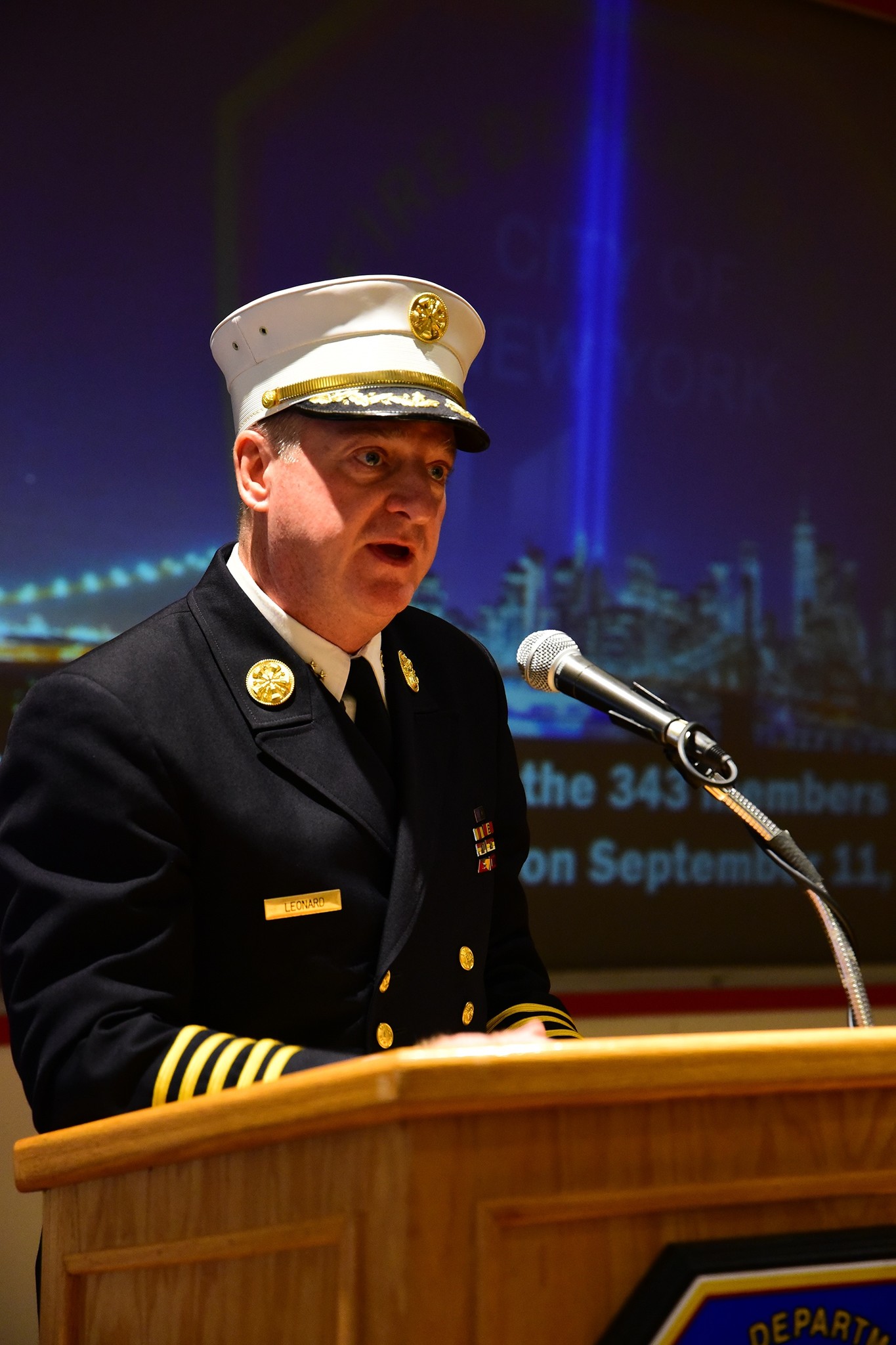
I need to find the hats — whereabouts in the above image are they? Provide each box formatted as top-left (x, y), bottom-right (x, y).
top-left (210, 275), bottom-right (491, 454)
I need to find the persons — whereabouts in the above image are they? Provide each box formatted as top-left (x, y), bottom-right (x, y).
top-left (0, 274), bottom-right (581, 1134)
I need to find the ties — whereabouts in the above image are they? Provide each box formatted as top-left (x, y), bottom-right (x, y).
top-left (345, 656), bottom-right (400, 793)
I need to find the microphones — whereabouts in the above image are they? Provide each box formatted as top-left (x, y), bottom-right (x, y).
top-left (516, 629), bottom-right (731, 769)
